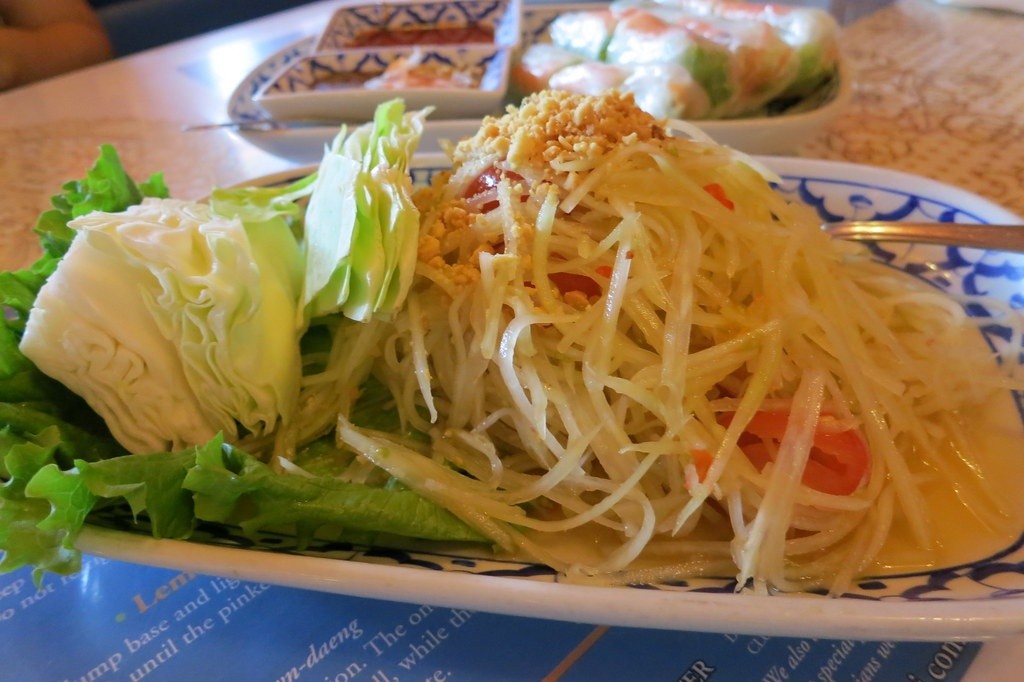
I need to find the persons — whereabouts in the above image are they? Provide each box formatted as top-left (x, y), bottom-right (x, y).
top-left (0, 0), bottom-right (113, 95)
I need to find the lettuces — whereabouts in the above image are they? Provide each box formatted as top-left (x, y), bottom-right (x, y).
top-left (0, 143), bottom-right (508, 588)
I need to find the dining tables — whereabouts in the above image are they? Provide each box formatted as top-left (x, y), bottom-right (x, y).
top-left (0, 0), bottom-right (1024, 681)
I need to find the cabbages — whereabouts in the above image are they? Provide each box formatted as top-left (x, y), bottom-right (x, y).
top-left (18, 97), bottom-right (440, 452)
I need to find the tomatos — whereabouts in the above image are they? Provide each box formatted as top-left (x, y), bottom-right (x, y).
top-left (693, 406), bottom-right (867, 499)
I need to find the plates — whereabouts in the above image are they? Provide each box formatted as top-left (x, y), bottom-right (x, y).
top-left (225, 6), bottom-right (850, 158)
top-left (249, 43), bottom-right (512, 127)
top-left (0, 150), bottom-right (1024, 639)
top-left (312, 1), bottom-right (524, 53)
